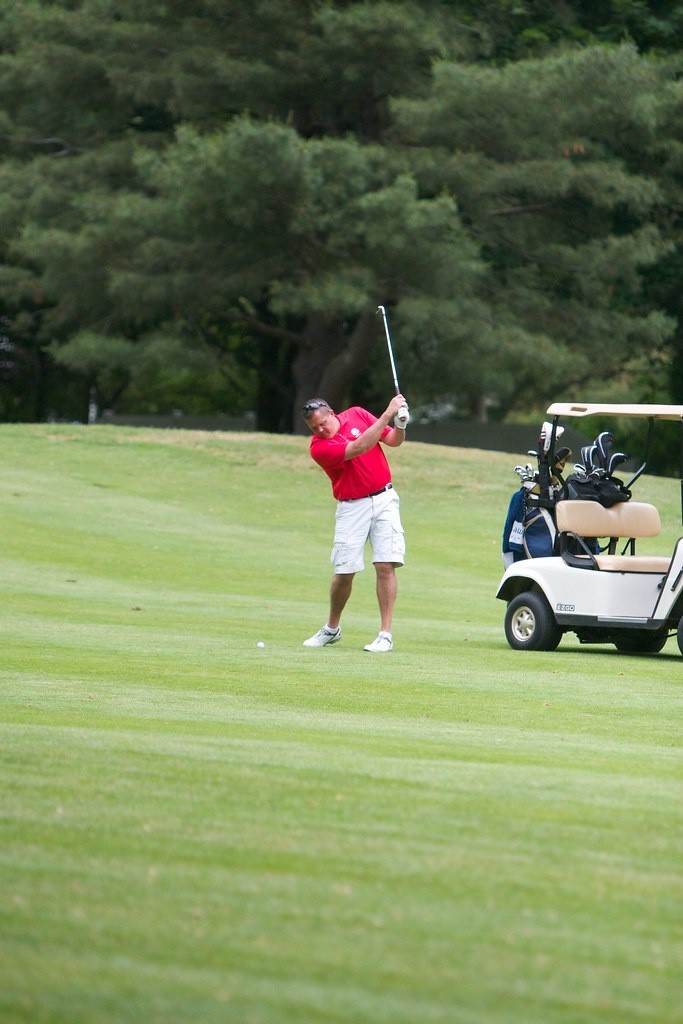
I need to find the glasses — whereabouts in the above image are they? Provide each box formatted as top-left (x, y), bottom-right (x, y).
top-left (301, 402), bottom-right (328, 416)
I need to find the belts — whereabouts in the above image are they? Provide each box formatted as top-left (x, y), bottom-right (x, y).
top-left (338, 484), bottom-right (392, 501)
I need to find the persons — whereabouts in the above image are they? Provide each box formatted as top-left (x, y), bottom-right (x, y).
top-left (301, 394), bottom-right (410, 652)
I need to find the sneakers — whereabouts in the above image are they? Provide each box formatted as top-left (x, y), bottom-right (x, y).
top-left (303, 624), bottom-right (342, 648)
top-left (363, 631), bottom-right (394, 652)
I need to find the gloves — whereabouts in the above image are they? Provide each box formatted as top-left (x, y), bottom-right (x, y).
top-left (394, 402), bottom-right (410, 430)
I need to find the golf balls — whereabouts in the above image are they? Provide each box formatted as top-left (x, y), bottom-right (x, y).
top-left (256, 642), bottom-right (265, 648)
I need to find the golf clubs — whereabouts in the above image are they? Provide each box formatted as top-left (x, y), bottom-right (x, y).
top-left (374, 305), bottom-right (405, 423)
top-left (513, 431), bottom-right (651, 489)
